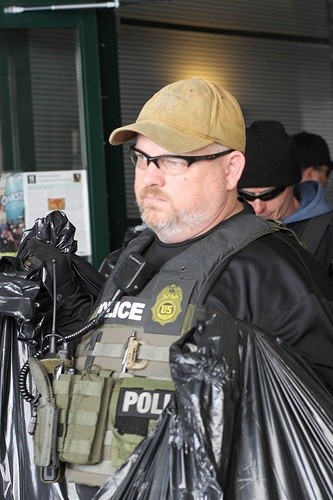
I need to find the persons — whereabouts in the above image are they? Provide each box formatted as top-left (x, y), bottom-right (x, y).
top-left (0, 215), bottom-right (25, 252)
top-left (18, 77), bottom-right (333, 500)
top-left (236, 120), bottom-right (333, 282)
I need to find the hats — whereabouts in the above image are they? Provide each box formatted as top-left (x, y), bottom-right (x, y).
top-left (108, 78), bottom-right (246, 155)
top-left (236, 120), bottom-right (302, 189)
top-left (289, 131), bottom-right (333, 169)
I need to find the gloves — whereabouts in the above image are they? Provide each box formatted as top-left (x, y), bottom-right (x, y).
top-left (26, 238), bottom-right (73, 289)
top-left (194, 304), bottom-right (240, 366)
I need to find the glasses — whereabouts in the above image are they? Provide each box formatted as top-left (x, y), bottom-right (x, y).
top-left (130, 143), bottom-right (236, 175)
top-left (237, 183), bottom-right (288, 201)
top-left (314, 165), bottom-right (331, 178)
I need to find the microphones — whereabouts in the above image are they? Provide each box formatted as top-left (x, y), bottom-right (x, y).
top-left (112, 251), bottom-right (152, 296)
